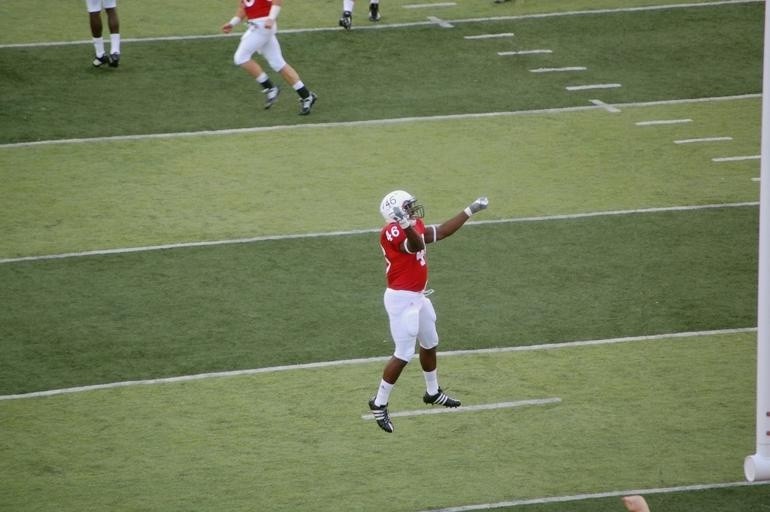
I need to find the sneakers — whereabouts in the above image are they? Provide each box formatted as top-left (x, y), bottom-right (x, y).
top-left (91, 53), bottom-right (119, 69)
top-left (370, 1), bottom-right (380, 21)
top-left (262, 86), bottom-right (279, 109)
top-left (423, 386), bottom-right (460, 408)
top-left (368, 396), bottom-right (394, 433)
top-left (339, 15), bottom-right (351, 30)
top-left (299, 92), bottom-right (317, 115)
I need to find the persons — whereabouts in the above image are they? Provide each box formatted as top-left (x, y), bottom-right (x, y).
top-left (621, 494), bottom-right (650, 512)
top-left (85, 0), bottom-right (121, 69)
top-left (338, 0), bottom-right (382, 31)
top-left (222, 1), bottom-right (319, 117)
top-left (368, 190), bottom-right (490, 432)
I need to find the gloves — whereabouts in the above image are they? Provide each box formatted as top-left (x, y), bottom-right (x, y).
top-left (393, 207), bottom-right (411, 229)
top-left (465, 198), bottom-right (488, 216)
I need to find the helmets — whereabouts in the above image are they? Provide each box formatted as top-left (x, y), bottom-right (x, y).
top-left (380, 190), bottom-right (424, 225)
